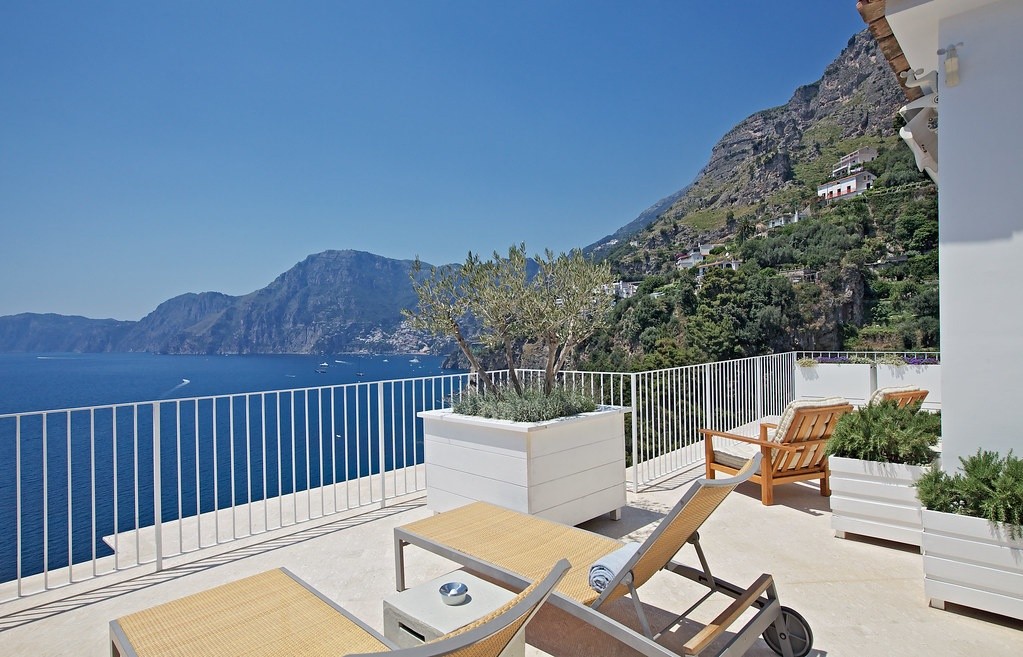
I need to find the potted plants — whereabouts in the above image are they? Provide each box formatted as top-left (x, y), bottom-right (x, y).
top-left (823, 397), bottom-right (941, 547)
top-left (911, 441), bottom-right (1023, 620)
top-left (399, 241), bottom-right (643, 529)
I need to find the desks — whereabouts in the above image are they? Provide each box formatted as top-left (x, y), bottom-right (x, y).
top-left (383, 569), bottom-right (525, 657)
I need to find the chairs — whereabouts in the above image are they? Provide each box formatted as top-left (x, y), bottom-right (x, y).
top-left (699, 396), bottom-right (854, 505)
top-left (868, 383), bottom-right (930, 414)
top-left (108, 452), bottom-right (814, 657)
